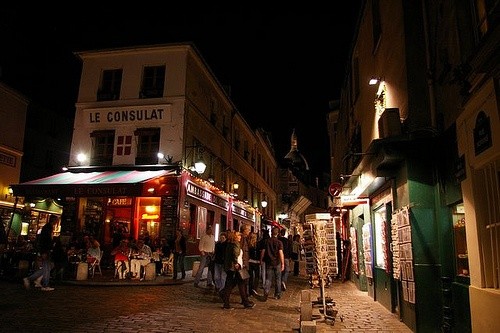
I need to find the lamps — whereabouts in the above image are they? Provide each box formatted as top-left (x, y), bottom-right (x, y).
top-left (368, 77), bottom-right (385, 85)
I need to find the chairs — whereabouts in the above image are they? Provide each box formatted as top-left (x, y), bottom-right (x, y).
top-left (89, 251), bottom-right (103, 279)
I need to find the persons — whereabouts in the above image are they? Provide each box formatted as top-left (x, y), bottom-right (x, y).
top-left (194, 222), bottom-right (302, 309)
top-left (0, 217), bottom-right (54, 288)
top-left (173, 229), bottom-right (187, 280)
top-left (49, 231), bottom-right (101, 278)
top-left (24, 215), bottom-right (59, 292)
top-left (110, 226), bottom-right (170, 280)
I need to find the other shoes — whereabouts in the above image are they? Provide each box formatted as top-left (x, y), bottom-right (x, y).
top-left (41, 287), bottom-right (54, 291)
top-left (251, 286), bottom-right (259, 295)
top-left (263, 291), bottom-right (269, 301)
top-left (245, 303), bottom-right (256, 309)
top-left (223, 305), bottom-right (234, 311)
top-left (194, 282), bottom-right (198, 286)
top-left (34, 281), bottom-right (42, 288)
top-left (207, 283), bottom-right (213, 287)
top-left (24, 278), bottom-right (29, 288)
top-left (281, 281), bottom-right (286, 291)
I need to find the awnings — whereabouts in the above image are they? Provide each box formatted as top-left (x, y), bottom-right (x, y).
top-left (8, 168), bottom-right (176, 197)
